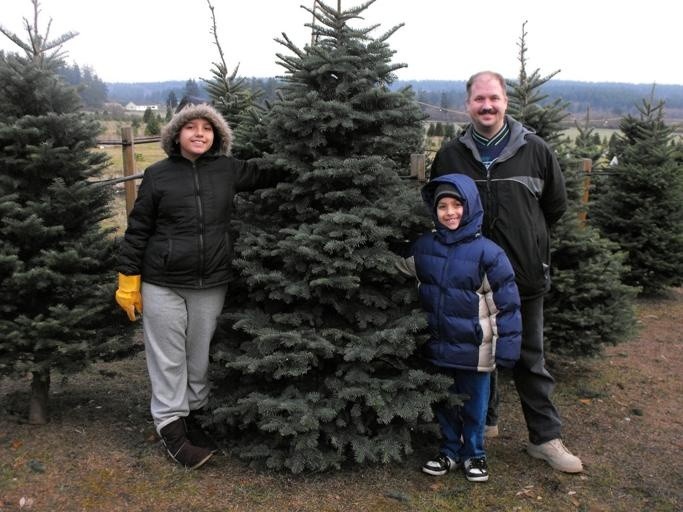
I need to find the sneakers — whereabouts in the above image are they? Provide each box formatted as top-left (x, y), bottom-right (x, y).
top-left (463, 455), bottom-right (490, 482)
top-left (526, 439), bottom-right (583, 474)
top-left (421, 455), bottom-right (461, 476)
top-left (460, 424), bottom-right (500, 445)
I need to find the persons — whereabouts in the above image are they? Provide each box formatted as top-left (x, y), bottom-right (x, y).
top-left (112, 97), bottom-right (290, 470)
top-left (428, 70), bottom-right (585, 476)
top-left (382, 173), bottom-right (522, 484)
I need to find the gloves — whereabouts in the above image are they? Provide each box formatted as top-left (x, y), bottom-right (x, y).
top-left (115, 270), bottom-right (143, 322)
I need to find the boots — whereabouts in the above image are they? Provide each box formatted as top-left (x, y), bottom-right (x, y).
top-left (155, 407), bottom-right (222, 471)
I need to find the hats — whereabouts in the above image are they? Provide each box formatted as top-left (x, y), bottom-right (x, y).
top-left (433, 182), bottom-right (464, 209)
